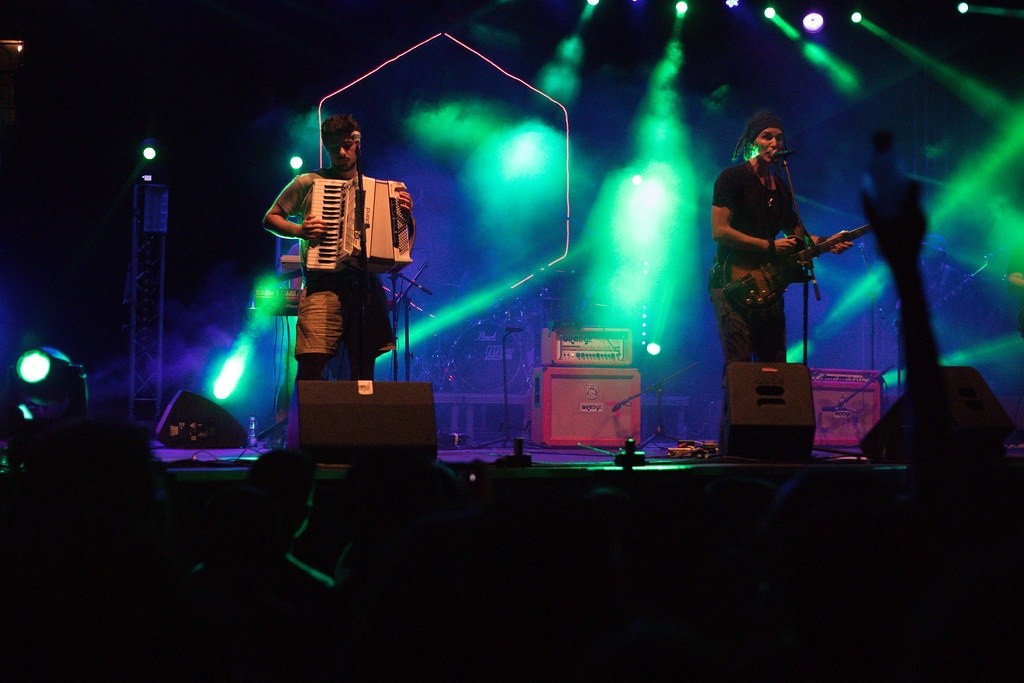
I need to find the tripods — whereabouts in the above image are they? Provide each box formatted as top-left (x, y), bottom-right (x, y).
top-left (620, 360), bottom-right (700, 452)
top-left (472, 326), bottom-right (555, 449)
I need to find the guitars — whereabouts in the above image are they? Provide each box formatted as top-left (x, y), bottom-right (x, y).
top-left (720, 223), bottom-right (873, 308)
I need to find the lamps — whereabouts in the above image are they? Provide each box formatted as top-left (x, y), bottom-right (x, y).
top-left (139, 138), bottom-right (164, 162)
top-left (7, 346), bottom-right (89, 417)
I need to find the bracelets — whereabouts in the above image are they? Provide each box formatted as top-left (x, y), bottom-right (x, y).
top-left (767, 238), bottom-right (775, 252)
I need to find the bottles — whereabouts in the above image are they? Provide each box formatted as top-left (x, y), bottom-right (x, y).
top-left (247, 416), bottom-right (257, 447)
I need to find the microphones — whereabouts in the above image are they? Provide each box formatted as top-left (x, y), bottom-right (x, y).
top-left (821, 405), bottom-right (848, 411)
top-left (612, 404), bottom-right (621, 412)
top-left (351, 130), bottom-right (361, 144)
top-left (396, 263), bottom-right (429, 305)
top-left (769, 149), bottom-right (796, 160)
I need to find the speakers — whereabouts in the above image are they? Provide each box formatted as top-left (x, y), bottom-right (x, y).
top-left (859, 366), bottom-right (1014, 465)
top-left (529, 365), bottom-right (642, 450)
top-left (809, 367), bottom-right (883, 446)
top-left (286, 380), bottom-right (438, 463)
top-left (154, 390), bottom-right (247, 449)
top-left (719, 362), bottom-right (817, 462)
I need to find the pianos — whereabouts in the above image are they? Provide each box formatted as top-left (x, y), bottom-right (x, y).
top-left (252, 286), bottom-right (405, 443)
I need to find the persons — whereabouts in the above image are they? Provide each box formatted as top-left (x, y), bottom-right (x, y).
top-left (707, 113), bottom-right (855, 459)
top-left (262, 114), bottom-right (417, 381)
